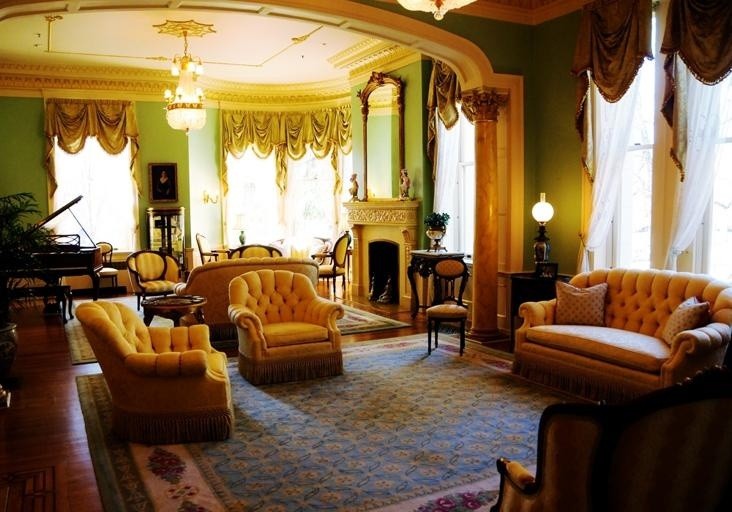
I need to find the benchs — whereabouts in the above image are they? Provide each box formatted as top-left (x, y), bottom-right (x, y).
top-left (7, 285), bottom-right (74, 324)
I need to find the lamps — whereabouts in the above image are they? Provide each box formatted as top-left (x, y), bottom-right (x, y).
top-left (532, 192), bottom-right (554, 279)
top-left (163, 27), bottom-right (207, 135)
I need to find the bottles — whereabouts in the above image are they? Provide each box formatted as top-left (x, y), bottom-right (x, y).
top-left (239, 231), bottom-right (245, 246)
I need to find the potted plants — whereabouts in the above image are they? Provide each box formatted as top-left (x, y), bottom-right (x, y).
top-left (424, 212), bottom-right (450, 239)
top-left (0, 191), bottom-right (62, 375)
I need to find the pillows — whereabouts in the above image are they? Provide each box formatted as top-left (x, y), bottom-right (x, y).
top-left (662, 296), bottom-right (710, 349)
top-left (555, 280), bottom-right (608, 326)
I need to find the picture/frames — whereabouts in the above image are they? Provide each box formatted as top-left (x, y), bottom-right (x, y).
top-left (149, 163), bottom-right (178, 203)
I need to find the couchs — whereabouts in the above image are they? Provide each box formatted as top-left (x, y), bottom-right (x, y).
top-left (511, 267), bottom-right (732, 400)
top-left (174, 257), bottom-right (319, 338)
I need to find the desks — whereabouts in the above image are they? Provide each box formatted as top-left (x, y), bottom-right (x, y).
top-left (407, 250), bottom-right (468, 319)
top-left (141, 295), bottom-right (208, 326)
top-left (499, 272), bottom-right (573, 346)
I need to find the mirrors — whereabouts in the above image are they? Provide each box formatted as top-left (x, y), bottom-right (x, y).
top-left (357, 72), bottom-right (407, 200)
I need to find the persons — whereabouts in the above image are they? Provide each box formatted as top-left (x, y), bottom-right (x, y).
top-left (349, 173), bottom-right (358, 201)
top-left (398, 168), bottom-right (411, 198)
top-left (154, 168), bottom-right (174, 199)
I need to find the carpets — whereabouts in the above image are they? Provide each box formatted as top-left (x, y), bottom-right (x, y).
top-left (76, 331), bottom-right (590, 512)
top-left (59, 295), bottom-right (411, 365)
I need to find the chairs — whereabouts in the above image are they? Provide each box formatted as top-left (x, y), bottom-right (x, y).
top-left (74, 300), bottom-right (234, 445)
top-left (196, 233), bottom-right (351, 292)
top-left (426, 256), bottom-right (470, 356)
top-left (490, 365), bottom-right (731, 512)
top-left (95, 242), bottom-right (118, 300)
top-left (125, 250), bottom-right (181, 311)
top-left (228, 269), bottom-right (344, 385)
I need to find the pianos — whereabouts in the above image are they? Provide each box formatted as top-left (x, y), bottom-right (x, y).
top-left (1, 194), bottom-right (104, 301)
